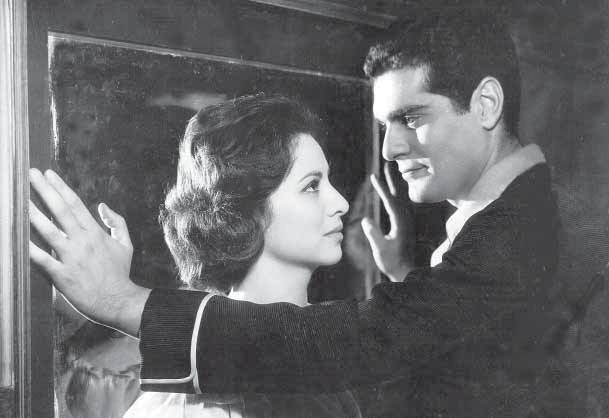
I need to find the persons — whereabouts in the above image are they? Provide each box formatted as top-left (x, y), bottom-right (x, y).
top-left (111, 91), bottom-right (350, 418)
top-left (27, 1), bottom-right (609, 417)
top-left (48, 99), bottom-right (359, 415)
